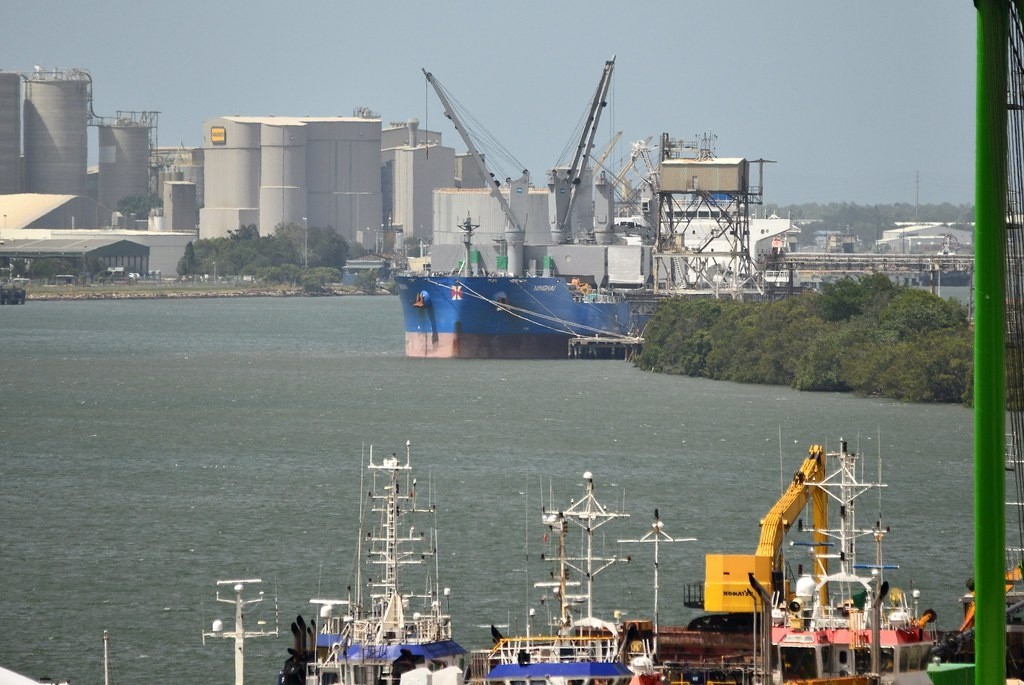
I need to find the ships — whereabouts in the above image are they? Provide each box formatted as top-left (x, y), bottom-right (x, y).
top-left (393, 49), bottom-right (788, 360)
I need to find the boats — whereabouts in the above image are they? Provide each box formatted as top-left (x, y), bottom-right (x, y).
top-left (214, 430), bottom-right (1024, 685)
